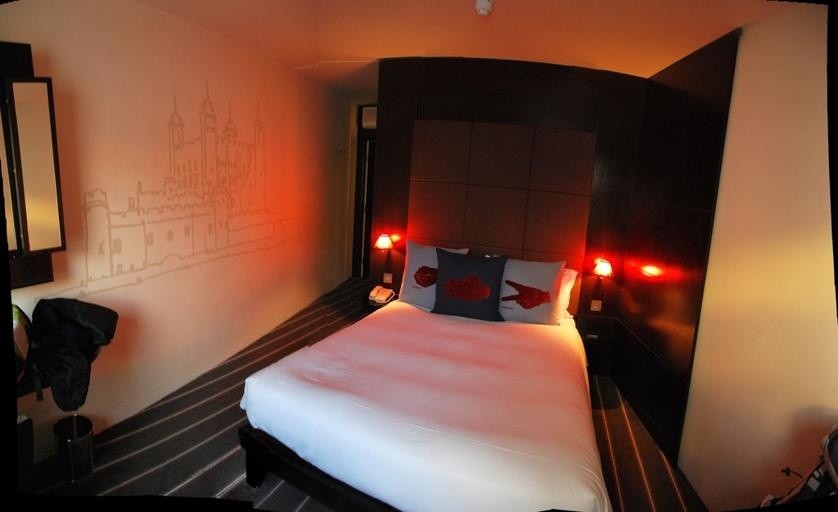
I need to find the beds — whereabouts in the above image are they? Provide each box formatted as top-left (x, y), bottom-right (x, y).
top-left (238, 298), bottom-right (613, 512)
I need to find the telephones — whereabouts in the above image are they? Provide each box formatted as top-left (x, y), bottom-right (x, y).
top-left (369, 285), bottom-right (395, 303)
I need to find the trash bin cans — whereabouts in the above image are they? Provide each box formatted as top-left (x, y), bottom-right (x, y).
top-left (53, 414), bottom-right (95, 479)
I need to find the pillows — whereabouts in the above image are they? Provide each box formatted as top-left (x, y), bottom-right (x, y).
top-left (398, 240), bottom-right (578, 326)
